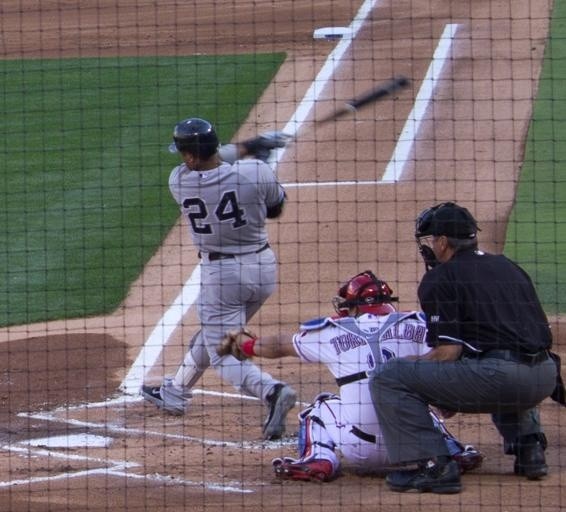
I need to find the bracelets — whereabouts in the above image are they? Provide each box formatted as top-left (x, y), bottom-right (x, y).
top-left (242, 339), bottom-right (256, 357)
top-left (215, 143), bottom-right (238, 164)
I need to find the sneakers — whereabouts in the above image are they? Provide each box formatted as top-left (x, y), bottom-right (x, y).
top-left (385, 460), bottom-right (461, 492)
top-left (263, 384), bottom-right (296, 439)
top-left (275, 459), bottom-right (332, 483)
top-left (139, 385), bottom-right (184, 416)
top-left (514, 444), bottom-right (547, 477)
top-left (454, 449), bottom-right (483, 473)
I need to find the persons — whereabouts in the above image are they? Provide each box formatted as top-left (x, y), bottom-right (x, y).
top-left (368, 202), bottom-right (557, 493)
top-left (218, 271), bottom-right (484, 481)
top-left (138, 118), bottom-right (297, 441)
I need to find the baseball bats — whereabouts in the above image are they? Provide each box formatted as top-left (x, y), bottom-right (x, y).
top-left (292, 76), bottom-right (409, 140)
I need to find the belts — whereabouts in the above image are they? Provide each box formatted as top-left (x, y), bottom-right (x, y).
top-left (351, 426), bottom-right (375, 443)
top-left (485, 352), bottom-right (548, 362)
top-left (199, 243), bottom-right (269, 259)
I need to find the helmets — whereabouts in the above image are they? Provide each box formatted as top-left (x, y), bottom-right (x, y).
top-left (331, 271), bottom-right (398, 316)
top-left (168, 118), bottom-right (218, 153)
top-left (414, 201), bottom-right (481, 271)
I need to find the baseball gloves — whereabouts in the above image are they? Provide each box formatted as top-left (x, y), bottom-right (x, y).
top-left (216, 329), bottom-right (257, 361)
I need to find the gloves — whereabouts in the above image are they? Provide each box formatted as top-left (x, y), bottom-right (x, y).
top-left (245, 132), bottom-right (291, 160)
